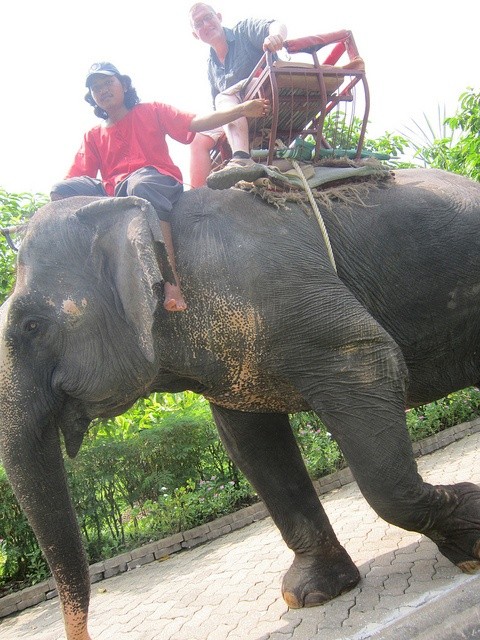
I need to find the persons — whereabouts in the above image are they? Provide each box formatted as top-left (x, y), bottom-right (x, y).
top-left (188, 2), bottom-right (287, 190)
top-left (50, 62), bottom-right (272, 311)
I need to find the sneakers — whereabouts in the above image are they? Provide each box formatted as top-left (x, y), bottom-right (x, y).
top-left (205, 157), bottom-right (264, 190)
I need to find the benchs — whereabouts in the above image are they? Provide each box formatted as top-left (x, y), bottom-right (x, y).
top-left (208, 30), bottom-right (372, 172)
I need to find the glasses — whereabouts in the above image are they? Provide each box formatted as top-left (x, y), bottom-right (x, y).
top-left (192, 13), bottom-right (213, 29)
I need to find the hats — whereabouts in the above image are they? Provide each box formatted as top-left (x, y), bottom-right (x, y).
top-left (86, 62), bottom-right (121, 88)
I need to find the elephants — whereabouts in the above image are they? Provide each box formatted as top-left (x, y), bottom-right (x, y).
top-left (0, 167), bottom-right (480, 640)
